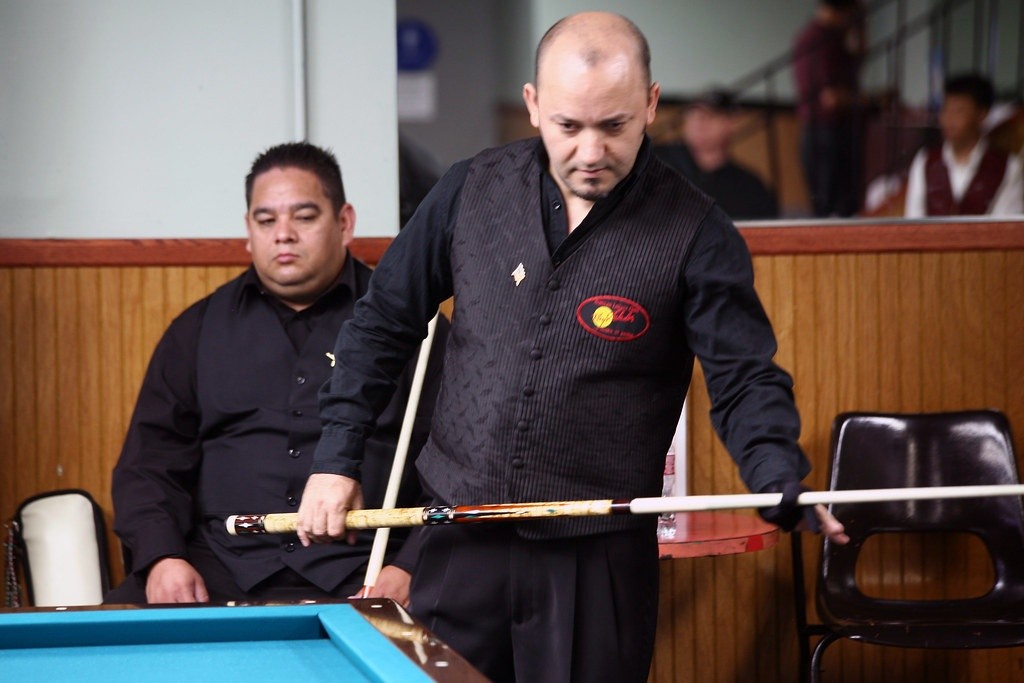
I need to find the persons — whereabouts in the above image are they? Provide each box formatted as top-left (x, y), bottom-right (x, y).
top-left (98, 143), bottom-right (452, 613)
top-left (863, 70), bottom-right (1024, 220)
top-left (659, 91), bottom-right (780, 220)
top-left (793, 0), bottom-right (885, 218)
top-left (294, 12), bottom-right (850, 683)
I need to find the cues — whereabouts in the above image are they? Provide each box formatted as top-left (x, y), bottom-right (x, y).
top-left (359, 306), bottom-right (442, 598)
top-left (223, 483), bottom-right (1024, 537)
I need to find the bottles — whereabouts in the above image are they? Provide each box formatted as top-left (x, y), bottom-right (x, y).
top-left (658, 439), bottom-right (675, 522)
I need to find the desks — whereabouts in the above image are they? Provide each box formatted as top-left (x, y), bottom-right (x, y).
top-left (658, 511), bottom-right (782, 560)
top-left (0, 597), bottom-right (491, 683)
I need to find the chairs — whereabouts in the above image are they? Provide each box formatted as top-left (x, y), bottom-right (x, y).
top-left (807, 407), bottom-right (1024, 683)
